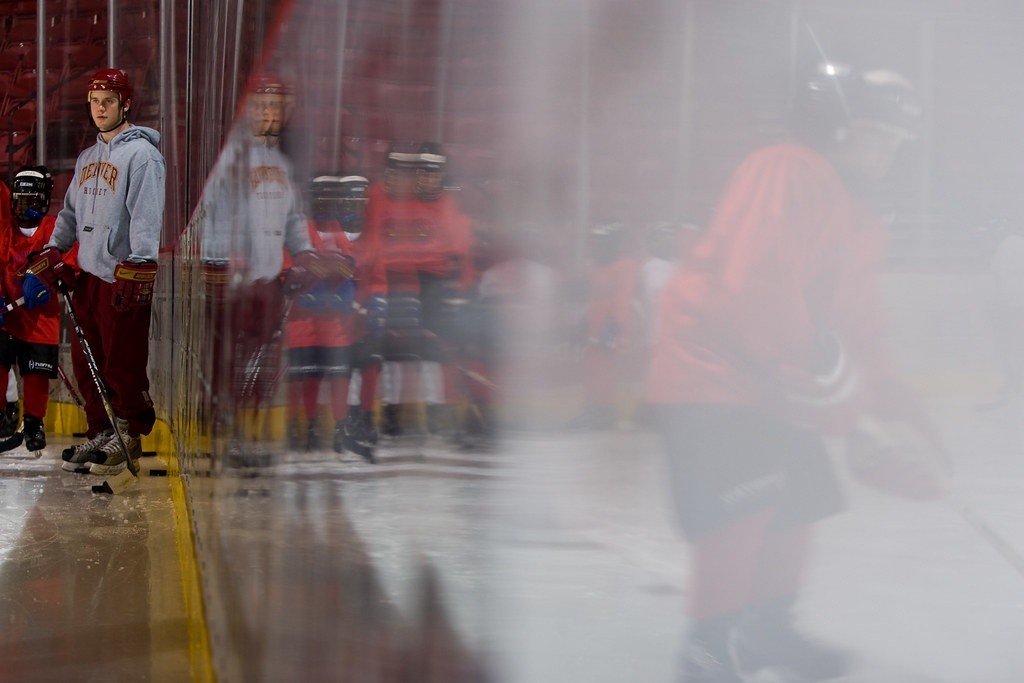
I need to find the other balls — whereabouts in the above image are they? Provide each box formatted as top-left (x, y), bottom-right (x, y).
top-left (72, 433), bottom-right (86, 437)
top-left (141, 451), bottom-right (157, 457)
top-left (74, 466), bottom-right (90, 474)
top-left (92, 485), bottom-right (105, 493)
top-left (150, 470), bottom-right (167, 476)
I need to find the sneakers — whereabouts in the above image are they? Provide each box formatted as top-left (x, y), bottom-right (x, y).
top-left (23, 414), bottom-right (47, 459)
top-left (61, 426), bottom-right (117, 472)
top-left (0, 403), bottom-right (24, 453)
top-left (88, 416), bottom-right (143, 476)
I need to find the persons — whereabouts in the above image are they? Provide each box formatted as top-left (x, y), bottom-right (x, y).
top-left (367, 140), bottom-right (497, 465)
top-left (27, 66), bottom-right (169, 476)
top-left (287, 166), bottom-right (385, 463)
top-left (0, 165), bottom-right (82, 452)
top-left (641, 61), bottom-right (961, 680)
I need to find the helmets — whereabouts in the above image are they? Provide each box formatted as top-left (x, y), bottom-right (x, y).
top-left (243, 66), bottom-right (287, 96)
top-left (86, 67), bottom-right (134, 107)
top-left (10, 165), bottom-right (55, 227)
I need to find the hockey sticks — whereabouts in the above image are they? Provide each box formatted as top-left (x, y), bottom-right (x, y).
top-left (55, 276), bottom-right (139, 495)
top-left (0, 289), bottom-right (83, 408)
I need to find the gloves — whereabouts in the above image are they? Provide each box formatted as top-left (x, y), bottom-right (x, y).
top-left (22, 269), bottom-right (50, 309)
top-left (0, 295), bottom-right (8, 326)
top-left (110, 260), bottom-right (159, 317)
top-left (27, 246), bottom-right (78, 293)
